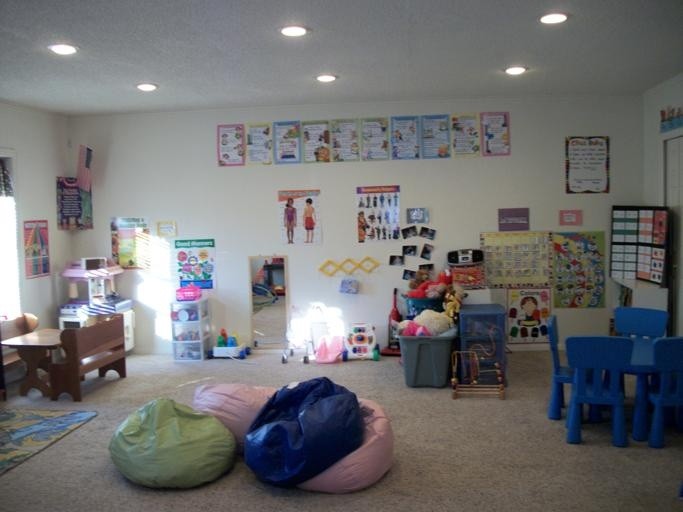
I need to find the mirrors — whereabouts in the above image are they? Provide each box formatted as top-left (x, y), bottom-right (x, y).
top-left (248, 255), bottom-right (289, 350)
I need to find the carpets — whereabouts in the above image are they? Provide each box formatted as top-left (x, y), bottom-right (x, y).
top-left (1, 410), bottom-right (99, 478)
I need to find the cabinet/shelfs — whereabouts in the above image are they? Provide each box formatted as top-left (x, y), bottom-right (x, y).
top-left (170, 295), bottom-right (210, 362)
top-left (459, 304), bottom-right (507, 384)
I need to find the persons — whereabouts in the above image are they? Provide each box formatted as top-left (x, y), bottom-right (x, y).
top-left (357, 210), bottom-right (369, 243)
top-left (301, 197), bottom-right (318, 244)
top-left (281, 196), bottom-right (298, 244)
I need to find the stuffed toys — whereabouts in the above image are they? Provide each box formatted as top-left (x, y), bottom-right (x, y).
top-left (397, 310), bottom-right (456, 339)
top-left (403, 267), bottom-right (453, 300)
top-left (408, 268), bottom-right (430, 290)
top-left (442, 287), bottom-right (468, 319)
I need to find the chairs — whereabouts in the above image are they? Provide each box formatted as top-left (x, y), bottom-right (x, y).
top-left (546, 307), bottom-right (682, 449)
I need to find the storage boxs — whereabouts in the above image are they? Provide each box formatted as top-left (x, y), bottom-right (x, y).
top-left (397, 336), bottom-right (454, 388)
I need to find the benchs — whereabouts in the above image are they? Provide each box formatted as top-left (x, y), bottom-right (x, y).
top-left (0, 313), bottom-right (126, 402)
